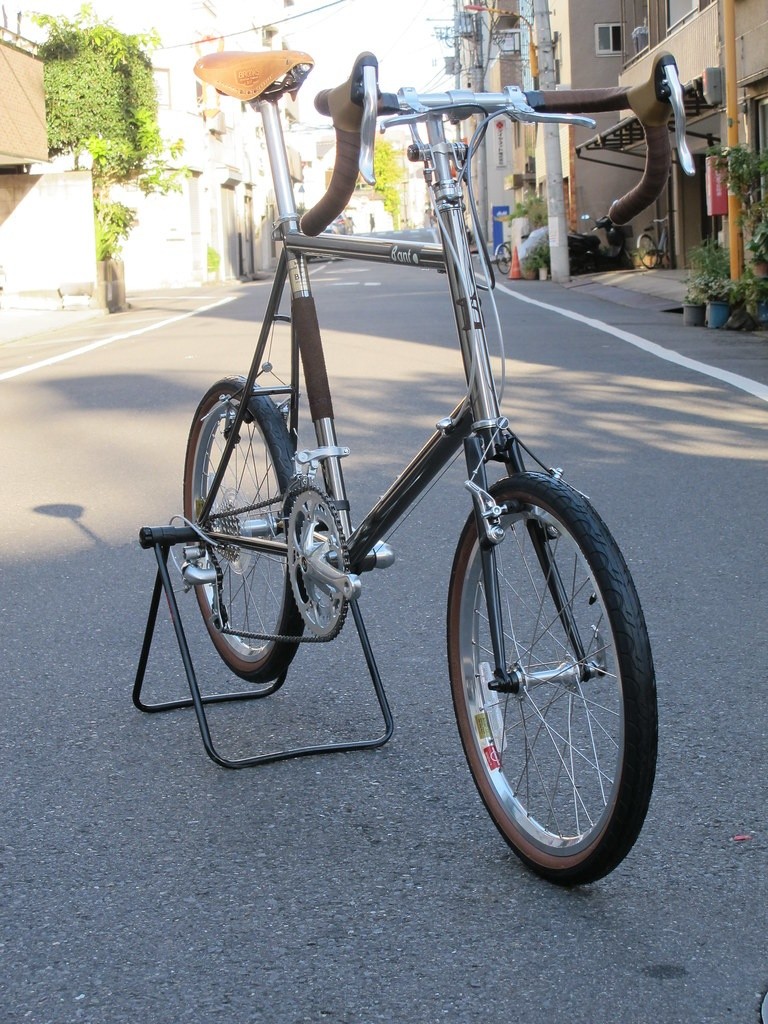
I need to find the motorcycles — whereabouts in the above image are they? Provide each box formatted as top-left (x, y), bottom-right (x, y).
top-left (568, 199), bottom-right (634, 275)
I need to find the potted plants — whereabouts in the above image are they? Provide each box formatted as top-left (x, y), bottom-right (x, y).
top-left (701, 277), bottom-right (730, 328)
top-left (681, 280), bottom-right (706, 327)
top-left (523, 258), bottom-right (538, 279)
top-left (739, 279), bottom-right (768, 322)
top-left (536, 256), bottom-right (548, 279)
top-left (750, 251), bottom-right (768, 278)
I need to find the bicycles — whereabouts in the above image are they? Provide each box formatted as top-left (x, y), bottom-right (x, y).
top-left (129, 49), bottom-right (698, 889)
top-left (636, 216), bottom-right (670, 270)
top-left (495, 233), bottom-right (513, 274)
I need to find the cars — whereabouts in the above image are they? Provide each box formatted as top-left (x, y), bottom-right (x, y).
top-left (304, 213), bottom-right (357, 261)
